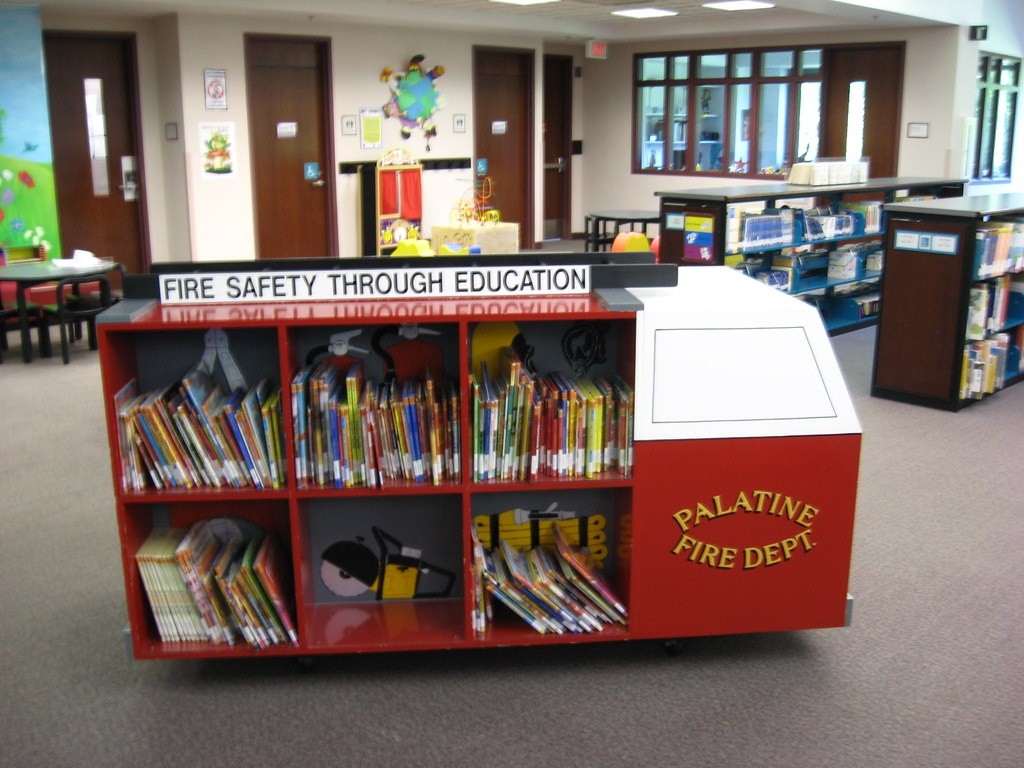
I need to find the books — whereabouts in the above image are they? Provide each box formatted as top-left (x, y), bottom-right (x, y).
top-left (112, 346), bottom-right (638, 650)
top-left (725, 201), bottom-right (1024, 400)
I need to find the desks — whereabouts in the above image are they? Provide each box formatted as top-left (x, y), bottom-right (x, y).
top-left (584, 211), bottom-right (660, 252)
top-left (0, 259), bottom-right (118, 363)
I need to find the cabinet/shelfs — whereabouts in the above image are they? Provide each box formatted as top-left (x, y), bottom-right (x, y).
top-left (646, 114), bottom-right (722, 144)
top-left (653, 176), bottom-right (968, 338)
top-left (93, 299), bottom-right (636, 660)
top-left (870, 189), bottom-right (1024, 413)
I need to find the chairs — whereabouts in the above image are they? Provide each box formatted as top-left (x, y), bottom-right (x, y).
top-left (0, 263), bottom-right (127, 364)
